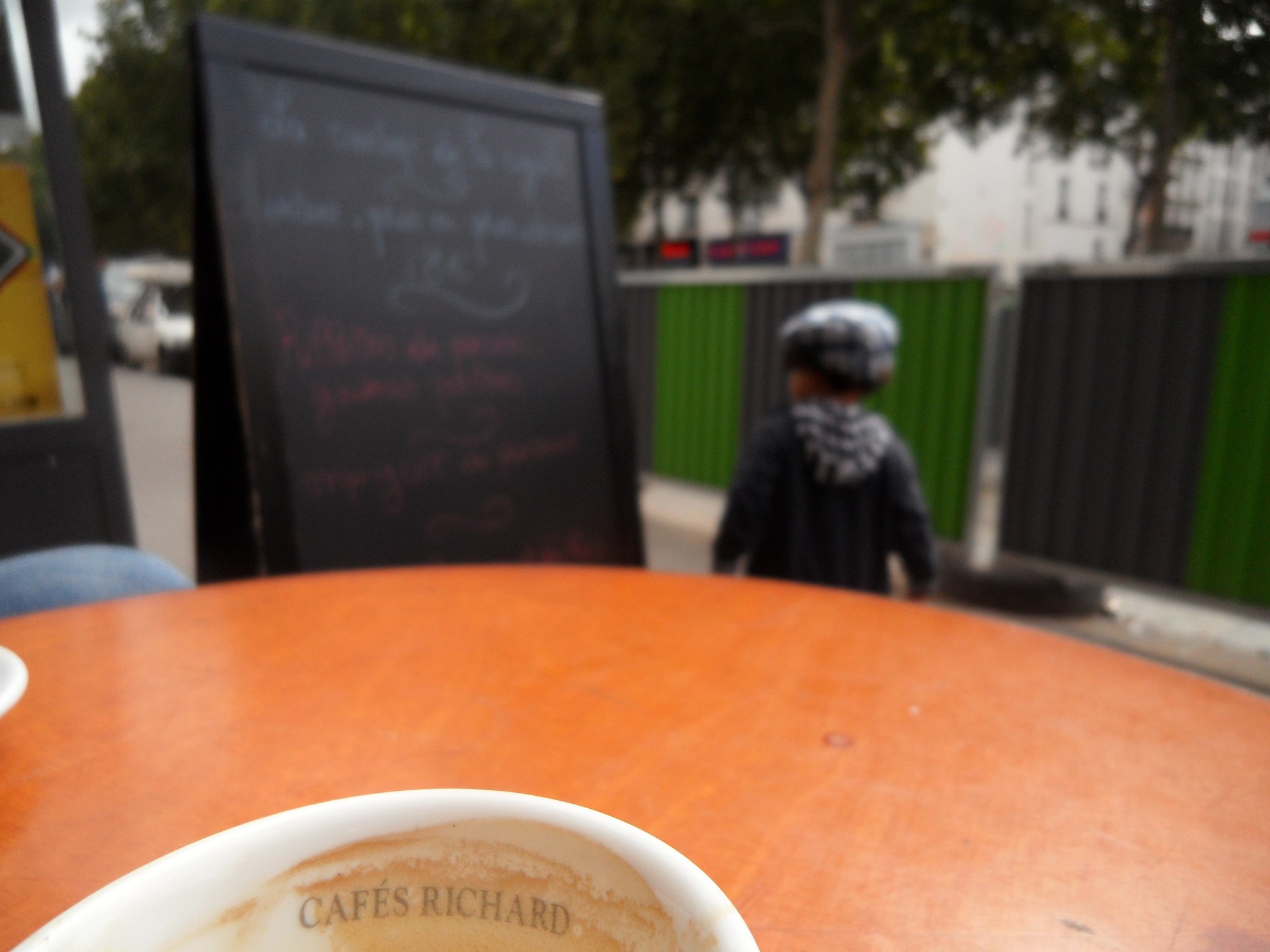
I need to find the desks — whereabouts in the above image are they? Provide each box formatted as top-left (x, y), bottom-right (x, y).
top-left (0, 560), bottom-right (1270, 952)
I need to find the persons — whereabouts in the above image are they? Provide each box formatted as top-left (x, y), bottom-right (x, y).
top-left (709, 296), bottom-right (933, 601)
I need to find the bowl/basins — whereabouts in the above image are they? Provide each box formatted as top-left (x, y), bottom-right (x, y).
top-left (13, 789), bottom-right (760, 952)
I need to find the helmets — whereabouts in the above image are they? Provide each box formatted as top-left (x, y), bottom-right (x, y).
top-left (776, 299), bottom-right (902, 392)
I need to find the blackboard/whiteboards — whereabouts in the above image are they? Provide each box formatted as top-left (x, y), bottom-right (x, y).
top-left (186, 18), bottom-right (648, 578)
